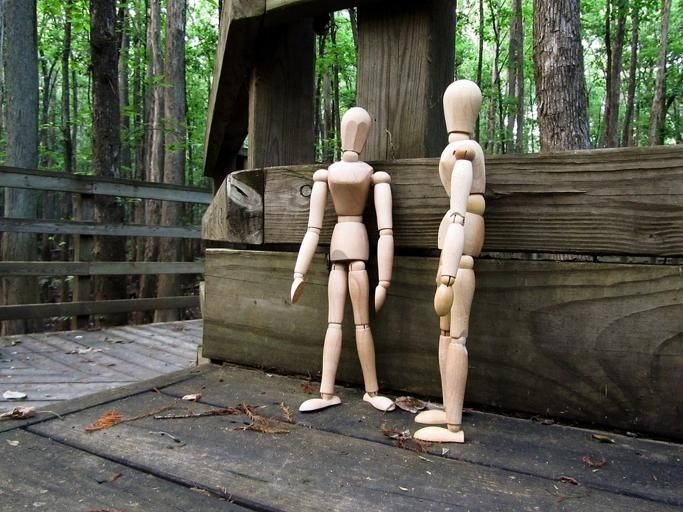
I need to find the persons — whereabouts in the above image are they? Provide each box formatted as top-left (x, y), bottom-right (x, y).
top-left (411, 78), bottom-right (486, 443)
top-left (289, 106), bottom-right (397, 413)
